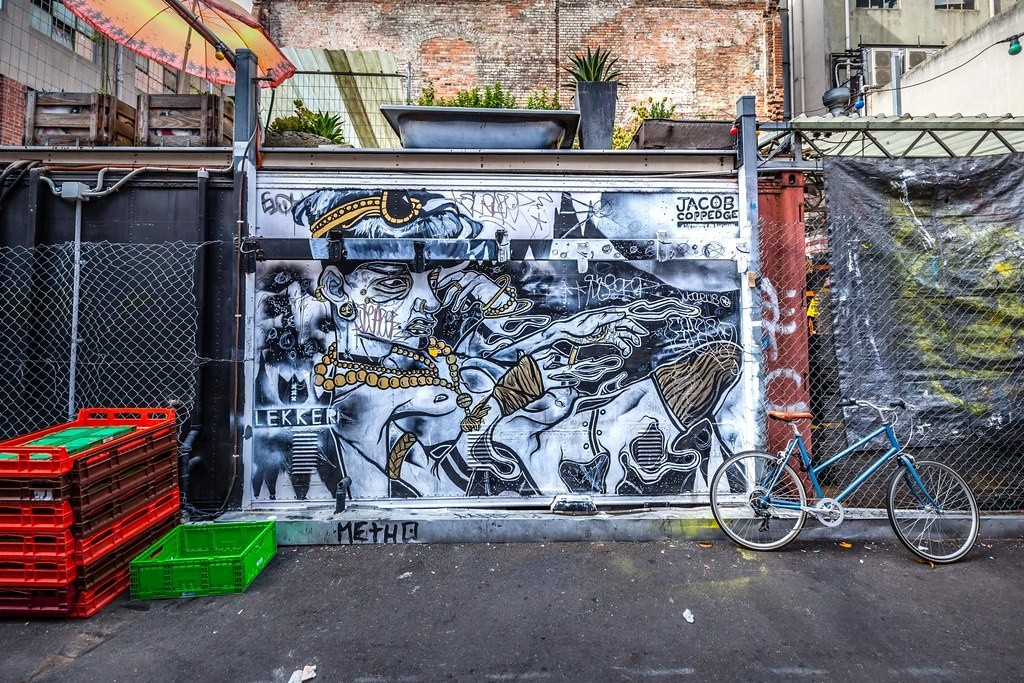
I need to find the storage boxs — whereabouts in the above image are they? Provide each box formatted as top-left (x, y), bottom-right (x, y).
top-left (134, 93), bottom-right (234, 147)
top-left (128, 520), bottom-right (277, 600)
top-left (0, 407), bottom-right (182, 621)
top-left (22, 90), bottom-right (137, 147)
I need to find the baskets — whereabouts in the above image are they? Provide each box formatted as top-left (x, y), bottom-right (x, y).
top-left (129, 519), bottom-right (279, 600)
top-left (0, 406), bottom-right (184, 618)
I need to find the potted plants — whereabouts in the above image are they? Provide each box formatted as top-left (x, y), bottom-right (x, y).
top-left (262, 97), bottom-right (350, 148)
top-left (380, 80), bottom-right (581, 150)
top-left (560, 46), bottom-right (627, 149)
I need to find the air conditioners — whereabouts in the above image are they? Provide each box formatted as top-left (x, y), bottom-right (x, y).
top-left (901, 48), bottom-right (926, 73)
top-left (868, 47), bottom-right (897, 88)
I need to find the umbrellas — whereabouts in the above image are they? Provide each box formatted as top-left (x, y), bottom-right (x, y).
top-left (63, 0), bottom-right (297, 92)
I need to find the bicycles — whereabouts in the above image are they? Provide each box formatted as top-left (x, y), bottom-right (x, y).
top-left (709, 397), bottom-right (981, 566)
